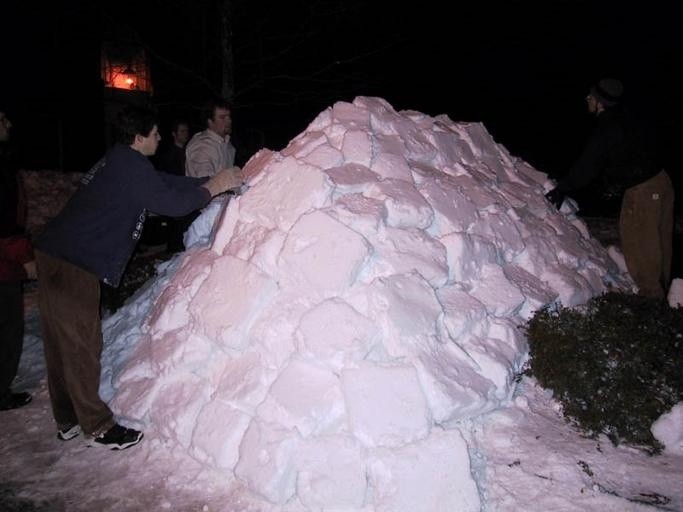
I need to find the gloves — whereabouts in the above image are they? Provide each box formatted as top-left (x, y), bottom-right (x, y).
top-left (543, 182), bottom-right (567, 212)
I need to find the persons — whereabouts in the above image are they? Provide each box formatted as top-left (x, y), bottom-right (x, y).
top-left (34, 100), bottom-right (244, 451)
top-left (546, 77), bottom-right (674, 305)
top-left (161, 118), bottom-right (191, 175)
top-left (183, 97), bottom-right (237, 176)
top-left (0, 108), bottom-right (33, 408)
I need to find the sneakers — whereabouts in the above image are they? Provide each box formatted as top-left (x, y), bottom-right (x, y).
top-left (80, 425), bottom-right (144, 452)
top-left (58, 421), bottom-right (82, 441)
top-left (1, 391), bottom-right (33, 411)
